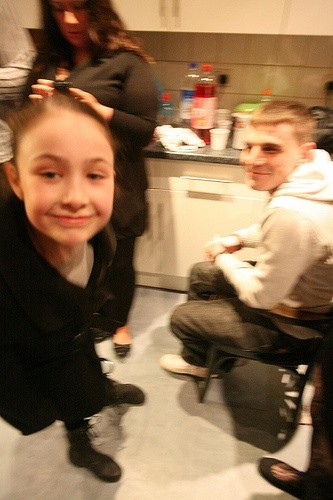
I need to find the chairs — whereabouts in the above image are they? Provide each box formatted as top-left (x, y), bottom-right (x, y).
top-left (199, 293), bottom-right (333, 429)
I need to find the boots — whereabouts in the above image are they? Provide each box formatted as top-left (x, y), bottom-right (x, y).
top-left (66, 429), bottom-right (121, 482)
top-left (102, 372), bottom-right (145, 404)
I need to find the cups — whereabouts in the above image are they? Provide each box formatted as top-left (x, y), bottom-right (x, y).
top-left (215, 109), bottom-right (230, 120)
top-left (208, 128), bottom-right (231, 151)
top-left (214, 120), bottom-right (234, 131)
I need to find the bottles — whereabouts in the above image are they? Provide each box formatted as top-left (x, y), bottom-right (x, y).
top-left (178, 62), bottom-right (201, 129)
top-left (259, 89), bottom-right (272, 108)
top-left (190, 64), bottom-right (219, 145)
top-left (160, 92), bottom-right (175, 125)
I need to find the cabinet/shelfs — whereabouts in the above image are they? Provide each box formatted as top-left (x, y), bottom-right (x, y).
top-left (109, 0), bottom-right (333, 37)
top-left (131, 158), bottom-right (272, 292)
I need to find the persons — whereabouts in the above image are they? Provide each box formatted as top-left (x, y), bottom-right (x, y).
top-left (0, 90), bottom-right (148, 483)
top-left (22, 0), bottom-right (159, 362)
top-left (257, 323), bottom-right (333, 500)
top-left (160, 100), bottom-right (333, 381)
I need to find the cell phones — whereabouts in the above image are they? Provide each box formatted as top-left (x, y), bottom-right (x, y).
top-left (54, 81), bottom-right (72, 96)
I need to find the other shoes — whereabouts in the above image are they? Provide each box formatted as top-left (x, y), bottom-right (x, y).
top-left (161, 353), bottom-right (209, 377)
top-left (114, 342), bottom-right (131, 356)
top-left (258, 457), bottom-right (310, 500)
